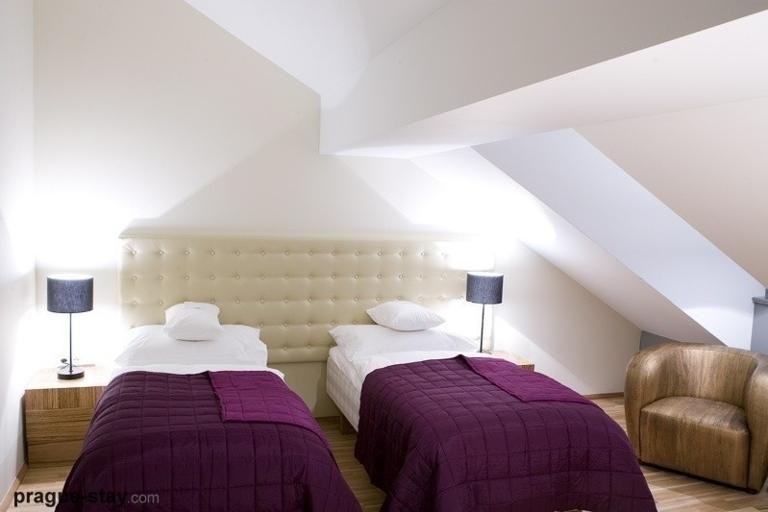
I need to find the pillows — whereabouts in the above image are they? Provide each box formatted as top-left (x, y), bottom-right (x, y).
top-left (123, 302), bottom-right (268, 372)
top-left (326, 324), bottom-right (481, 363)
top-left (365, 300), bottom-right (444, 332)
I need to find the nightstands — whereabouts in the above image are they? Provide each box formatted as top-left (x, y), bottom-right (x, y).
top-left (24, 366), bottom-right (114, 467)
top-left (486, 351), bottom-right (534, 373)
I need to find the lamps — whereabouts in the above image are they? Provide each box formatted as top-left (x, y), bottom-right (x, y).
top-left (47, 273), bottom-right (93, 380)
top-left (466, 272), bottom-right (504, 353)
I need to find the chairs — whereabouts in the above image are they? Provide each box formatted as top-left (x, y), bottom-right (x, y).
top-left (623, 343), bottom-right (768, 495)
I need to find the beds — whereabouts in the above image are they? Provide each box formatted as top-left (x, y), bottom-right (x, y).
top-left (324, 346), bottom-right (658, 511)
top-left (55, 365), bottom-right (363, 510)
